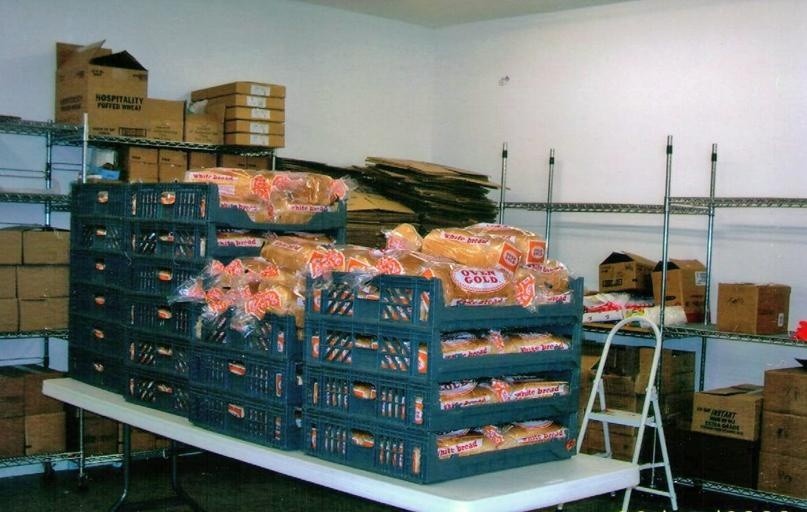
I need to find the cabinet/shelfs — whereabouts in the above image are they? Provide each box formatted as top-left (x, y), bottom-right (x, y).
top-left (492, 138), bottom-right (807, 510)
top-left (0, 106), bottom-right (92, 478)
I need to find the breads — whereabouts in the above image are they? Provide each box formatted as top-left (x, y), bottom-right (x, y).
top-left (182, 167), bottom-right (569, 473)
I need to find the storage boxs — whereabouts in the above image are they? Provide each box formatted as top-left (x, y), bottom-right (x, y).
top-left (63, 163), bottom-right (590, 486)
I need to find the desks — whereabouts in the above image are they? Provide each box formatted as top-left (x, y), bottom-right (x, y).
top-left (37, 372), bottom-right (643, 510)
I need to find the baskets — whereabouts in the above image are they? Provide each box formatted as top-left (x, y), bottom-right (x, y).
top-left (121, 255), bottom-right (204, 418)
top-left (68, 255), bottom-right (124, 394)
top-left (70, 181), bottom-right (347, 265)
top-left (187, 300), bottom-right (303, 451)
top-left (67, 253), bottom-right (584, 484)
